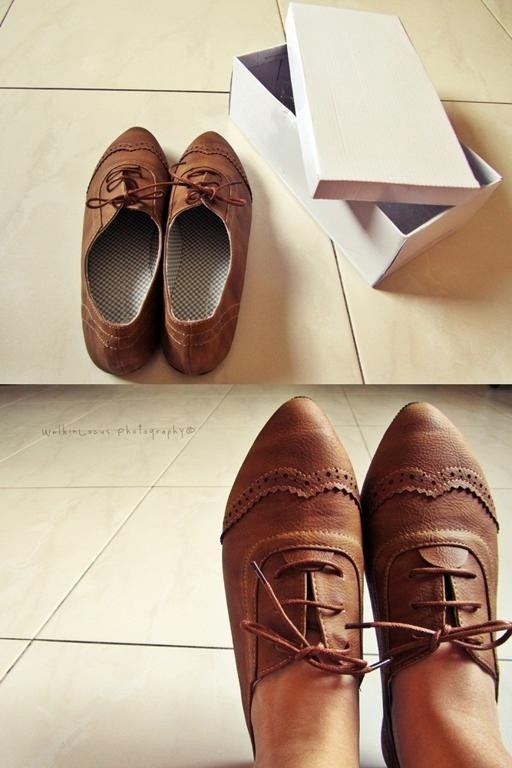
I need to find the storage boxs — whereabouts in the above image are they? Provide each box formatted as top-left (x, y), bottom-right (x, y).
top-left (232, 3), bottom-right (502, 292)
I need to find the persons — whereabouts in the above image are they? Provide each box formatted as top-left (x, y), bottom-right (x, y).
top-left (219, 395), bottom-right (512, 768)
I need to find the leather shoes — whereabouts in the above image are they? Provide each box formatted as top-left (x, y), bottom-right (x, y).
top-left (359, 401), bottom-right (511, 768)
top-left (79, 126), bottom-right (171, 378)
top-left (218, 394), bottom-right (366, 758)
top-left (161, 129), bottom-right (254, 379)
